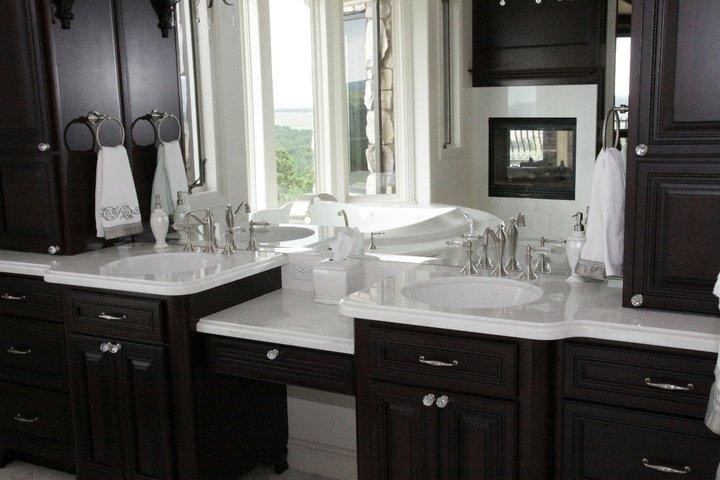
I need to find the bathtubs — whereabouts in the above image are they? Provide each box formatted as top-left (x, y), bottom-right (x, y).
top-left (248, 200), bottom-right (471, 235)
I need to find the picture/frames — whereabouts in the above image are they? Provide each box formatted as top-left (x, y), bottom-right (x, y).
top-left (488, 118), bottom-right (576, 201)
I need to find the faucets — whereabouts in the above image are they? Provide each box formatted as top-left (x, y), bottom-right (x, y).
top-left (184, 207), bottom-right (217, 254)
top-left (480, 224), bottom-right (509, 276)
top-left (224, 200), bottom-right (252, 248)
top-left (336, 210), bottom-right (349, 228)
top-left (506, 211), bottom-right (525, 271)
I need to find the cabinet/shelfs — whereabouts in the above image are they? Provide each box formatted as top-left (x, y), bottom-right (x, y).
top-left (355, 319), bottom-right (521, 478)
top-left (209, 337), bottom-right (355, 399)
top-left (1, 0), bottom-right (129, 255)
top-left (621, 1), bottom-right (719, 320)
top-left (471, 0), bottom-right (609, 90)
top-left (2, 273), bottom-right (77, 468)
top-left (562, 342), bottom-right (719, 479)
top-left (65, 289), bottom-right (177, 478)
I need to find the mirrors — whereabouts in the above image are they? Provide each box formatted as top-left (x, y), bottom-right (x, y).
top-left (115, 0), bottom-right (633, 279)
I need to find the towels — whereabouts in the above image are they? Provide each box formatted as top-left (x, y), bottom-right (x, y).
top-left (150, 140), bottom-right (192, 216)
top-left (575, 146), bottom-right (627, 280)
top-left (619, 149), bottom-right (626, 172)
top-left (93, 145), bottom-right (143, 241)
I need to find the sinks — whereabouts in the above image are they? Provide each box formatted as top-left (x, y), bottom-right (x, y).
top-left (99, 252), bottom-right (221, 273)
top-left (399, 277), bottom-right (544, 309)
top-left (218, 225), bottom-right (316, 244)
